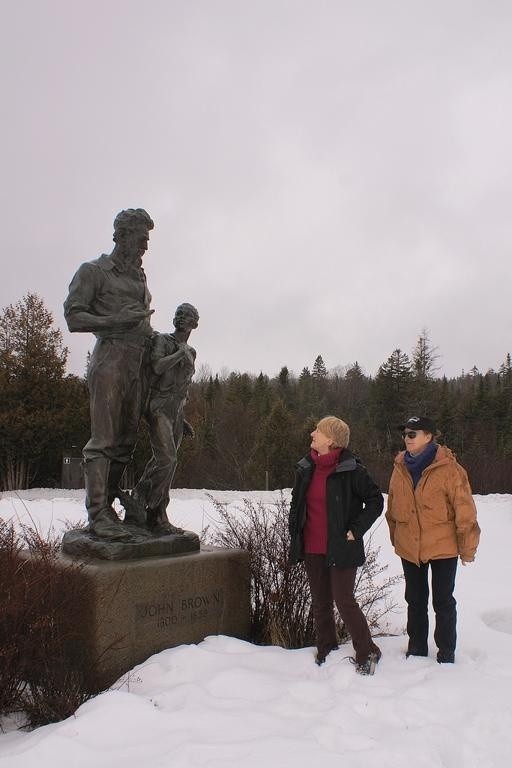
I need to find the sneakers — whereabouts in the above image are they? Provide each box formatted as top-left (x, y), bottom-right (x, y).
top-left (406, 652), bottom-right (455, 664)
top-left (315, 646), bottom-right (339, 666)
top-left (355, 645), bottom-right (382, 677)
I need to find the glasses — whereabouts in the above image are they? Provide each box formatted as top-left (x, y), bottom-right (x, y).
top-left (401, 431), bottom-right (424, 439)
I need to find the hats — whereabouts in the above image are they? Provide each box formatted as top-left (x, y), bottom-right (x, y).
top-left (397, 416), bottom-right (436, 435)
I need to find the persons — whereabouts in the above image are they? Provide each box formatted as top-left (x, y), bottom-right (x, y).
top-left (63, 206), bottom-right (154, 540)
top-left (286, 416), bottom-right (383, 676)
top-left (143, 302), bottom-right (200, 537)
top-left (384, 413), bottom-right (482, 664)
